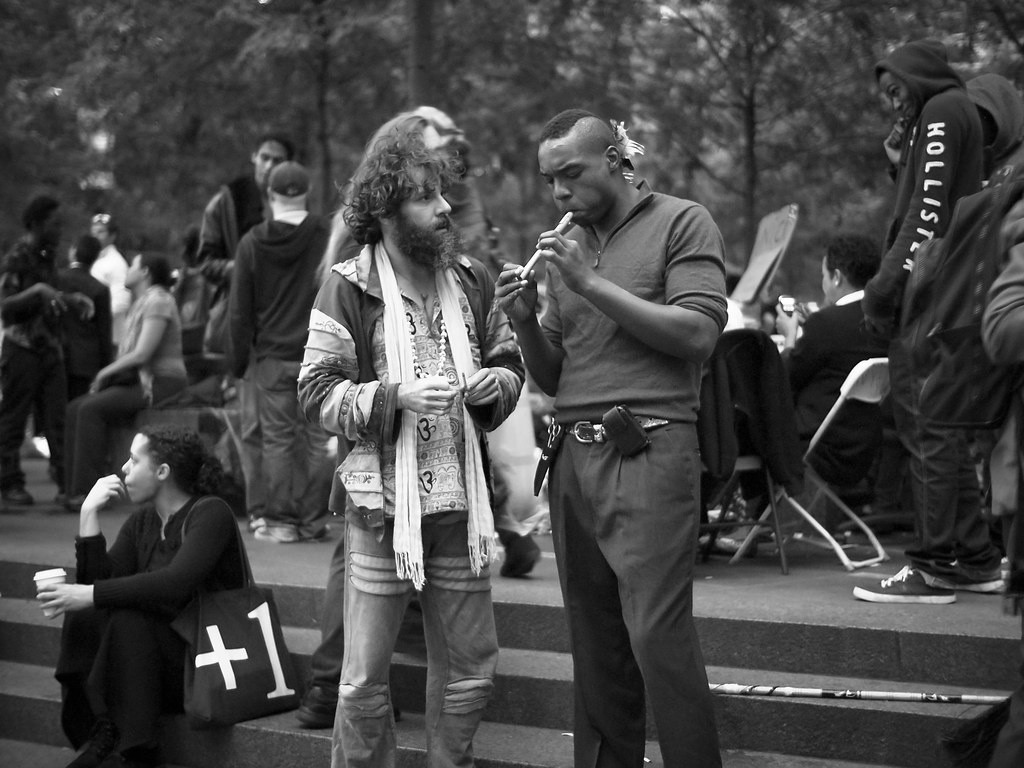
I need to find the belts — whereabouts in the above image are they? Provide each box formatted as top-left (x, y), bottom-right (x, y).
top-left (563, 414), bottom-right (671, 443)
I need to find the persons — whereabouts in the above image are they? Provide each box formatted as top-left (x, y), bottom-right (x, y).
top-left (35, 421), bottom-right (257, 761)
top-left (294, 105), bottom-right (495, 732)
top-left (980, 198), bottom-right (1024, 768)
top-left (296, 129), bottom-right (527, 768)
top-left (495, 106), bottom-right (727, 768)
top-left (0, 126), bottom-right (555, 576)
top-left (730, 233), bottom-right (887, 528)
top-left (964, 72), bottom-right (1023, 556)
top-left (849, 37), bottom-right (1005, 604)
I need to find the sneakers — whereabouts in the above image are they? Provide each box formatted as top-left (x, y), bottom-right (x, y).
top-left (851, 564), bottom-right (958, 603)
top-left (956, 564), bottom-right (1004, 593)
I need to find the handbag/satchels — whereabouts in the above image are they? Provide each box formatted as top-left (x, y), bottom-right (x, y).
top-left (170, 583), bottom-right (305, 730)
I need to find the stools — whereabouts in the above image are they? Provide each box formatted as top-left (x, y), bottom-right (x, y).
top-left (108, 406), bottom-right (237, 507)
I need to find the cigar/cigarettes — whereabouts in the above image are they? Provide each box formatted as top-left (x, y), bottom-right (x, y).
top-left (461, 372), bottom-right (469, 388)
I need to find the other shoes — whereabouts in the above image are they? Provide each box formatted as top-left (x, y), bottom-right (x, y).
top-left (2, 488), bottom-right (33, 505)
top-left (296, 685), bottom-right (401, 727)
top-left (249, 517), bottom-right (266, 531)
top-left (699, 534), bottom-right (758, 556)
top-left (254, 525), bottom-right (298, 544)
top-left (307, 524), bottom-right (331, 542)
top-left (65, 495), bottom-right (112, 511)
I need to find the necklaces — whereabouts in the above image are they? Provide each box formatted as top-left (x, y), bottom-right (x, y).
top-left (409, 317), bottom-right (450, 379)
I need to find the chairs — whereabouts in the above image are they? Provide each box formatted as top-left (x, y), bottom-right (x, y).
top-left (730, 356), bottom-right (892, 572)
top-left (693, 326), bottom-right (806, 574)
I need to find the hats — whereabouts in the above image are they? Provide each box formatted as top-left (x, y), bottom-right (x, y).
top-left (267, 160), bottom-right (309, 197)
